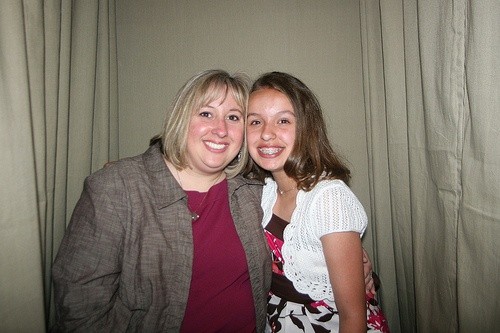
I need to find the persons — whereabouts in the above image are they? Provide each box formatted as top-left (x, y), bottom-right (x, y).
top-left (243, 72), bottom-right (392, 333)
top-left (47, 67), bottom-right (382, 333)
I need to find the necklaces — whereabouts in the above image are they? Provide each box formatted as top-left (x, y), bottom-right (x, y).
top-left (174, 165), bottom-right (220, 222)
top-left (276, 185), bottom-right (300, 194)
